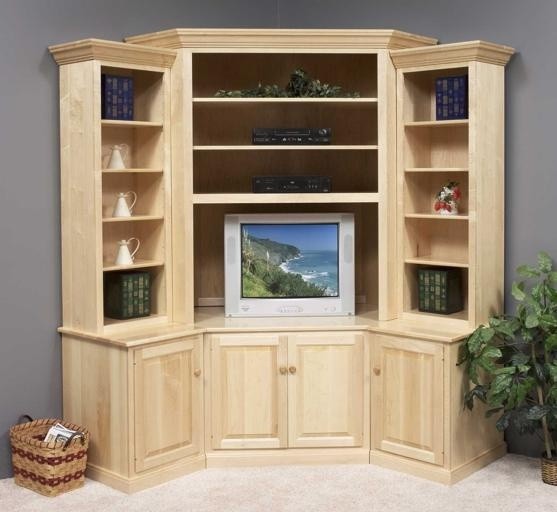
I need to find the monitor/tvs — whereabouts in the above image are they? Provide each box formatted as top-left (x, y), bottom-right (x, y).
top-left (224, 210), bottom-right (356, 319)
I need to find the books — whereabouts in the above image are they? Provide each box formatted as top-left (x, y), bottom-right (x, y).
top-left (44, 423), bottom-right (83, 444)
top-left (103, 73), bottom-right (133, 120)
top-left (418, 266), bottom-right (461, 313)
top-left (434, 75), bottom-right (467, 120)
top-left (108, 272), bottom-right (151, 319)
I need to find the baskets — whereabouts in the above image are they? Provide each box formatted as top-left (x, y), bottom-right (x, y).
top-left (541, 449), bottom-right (557, 487)
top-left (9, 413), bottom-right (86, 496)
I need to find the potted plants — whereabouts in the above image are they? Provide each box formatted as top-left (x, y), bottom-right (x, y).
top-left (455, 250), bottom-right (557, 486)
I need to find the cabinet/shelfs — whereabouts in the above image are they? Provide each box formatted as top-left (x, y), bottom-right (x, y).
top-left (49, 35), bottom-right (173, 331)
top-left (51, 332), bottom-right (202, 491)
top-left (365, 327), bottom-right (505, 485)
top-left (173, 29), bottom-right (386, 206)
top-left (385, 37), bottom-right (516, 328)
top-left (201, 332), bottom-right (366, 458)
